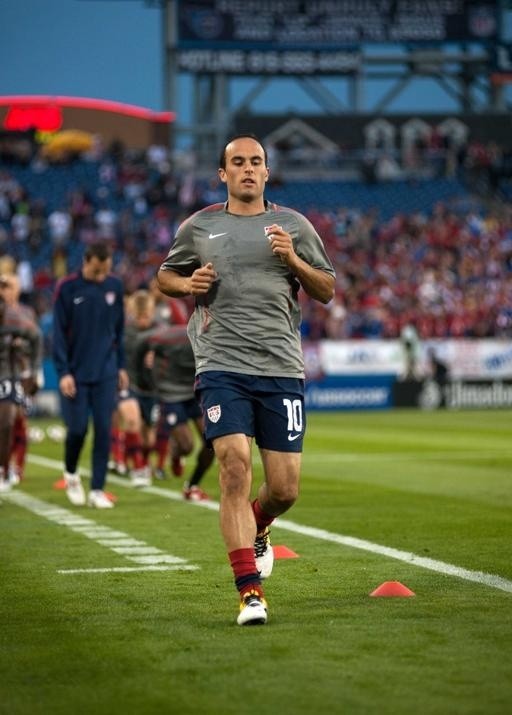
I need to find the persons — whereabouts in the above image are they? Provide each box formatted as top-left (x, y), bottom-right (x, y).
top-left (1, 123), bottom-right (222, 510)
top-left (157, 136), bottom-right (337, 628)
top-left (428, 349), bottom-right (451, 409)
top-left (295, 202), bottom-right (510, 342)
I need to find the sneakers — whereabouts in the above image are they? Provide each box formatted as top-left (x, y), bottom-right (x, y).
top-left (237, 593), bottom-right (268, 625)
top-left (88, 490), bottom-right (114, 510)
top-left (254, 527), bottom-right (273, 580)
top-left (63, 470), bottom-right (86, 506)
top-left (183, 481), bottom-right (209, 504)
